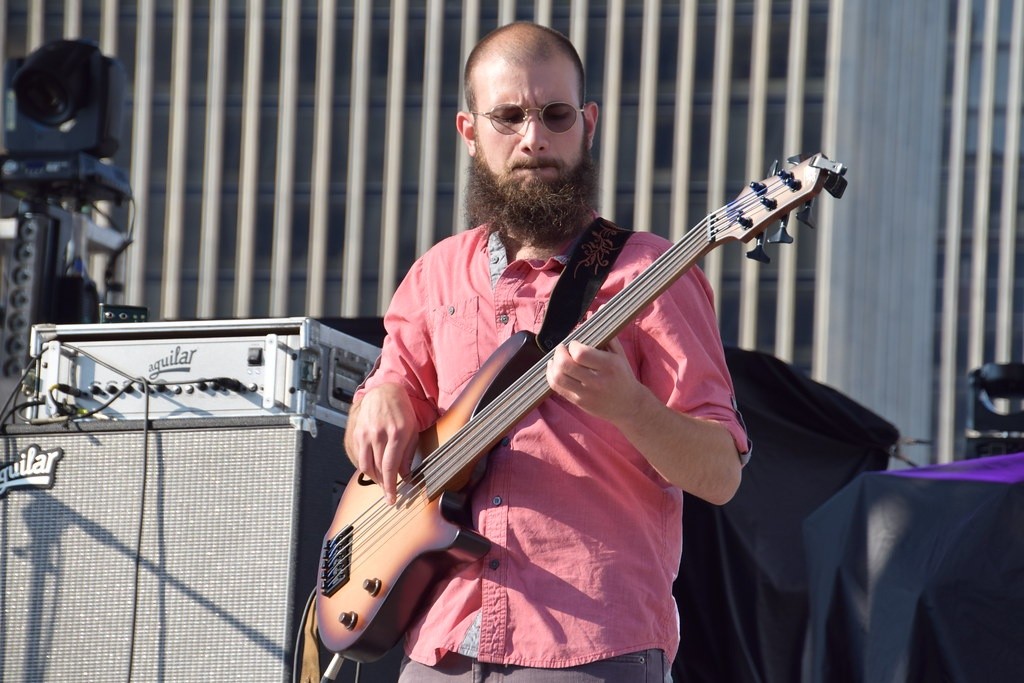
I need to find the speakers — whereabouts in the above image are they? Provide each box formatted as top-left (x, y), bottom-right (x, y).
top-left (1, 410), bottom-right (357, 680)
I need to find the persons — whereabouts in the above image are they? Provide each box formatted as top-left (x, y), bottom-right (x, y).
top-left (344, 20), bottom-right (752, 682)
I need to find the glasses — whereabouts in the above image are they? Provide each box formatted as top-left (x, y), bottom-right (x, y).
top-left (472, 102), bottom-right (587, 133)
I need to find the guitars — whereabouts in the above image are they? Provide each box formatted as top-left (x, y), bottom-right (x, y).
top-left (312, 150), bottom-right (849, 668)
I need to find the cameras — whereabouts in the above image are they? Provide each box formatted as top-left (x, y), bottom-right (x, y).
top-left (6, 38), bottom-right (125, 161)
